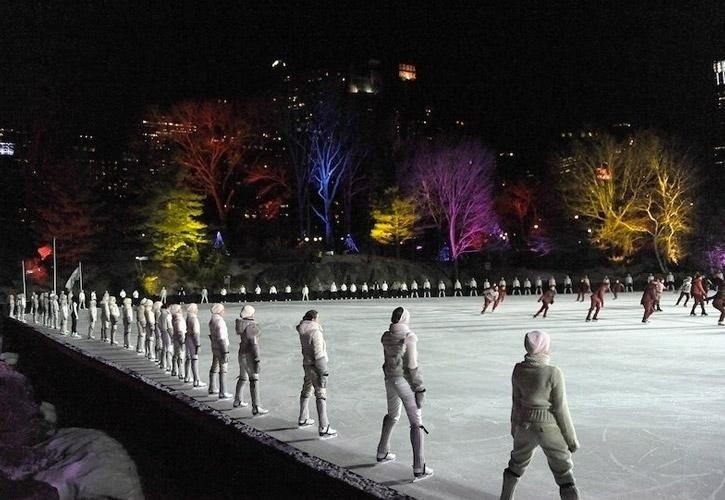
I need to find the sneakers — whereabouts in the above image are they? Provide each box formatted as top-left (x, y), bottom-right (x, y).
top-left (412, 466), bottom-right (433, 476)
top-left (209, 388), bottom-right (268, 415)
top-left (377, 452), bottom-right (397, 461)
top-left (71, 332), bottom-right (134, 351)
top-left (318, 425), bottom-right (336, 434)
top-left (137, 347), bottom-right (206, 387)
top-left (300, 419), bottom-right (316, 424)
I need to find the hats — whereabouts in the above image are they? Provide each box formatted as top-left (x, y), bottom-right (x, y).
top-left (8, 290), bottom-right (254, 321)
top-left (525, 332), bottom-right (550, 356)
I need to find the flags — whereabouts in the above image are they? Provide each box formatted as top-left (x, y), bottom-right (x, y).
top-left (38, 244), bottom-right (53, 258)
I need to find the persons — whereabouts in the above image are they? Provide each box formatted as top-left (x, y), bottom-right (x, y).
top-left (340, 271), bottom-right (721, 327)
top-left (234, 304), bottom-right (270, 416)
top-left (498, 330), bottom-right (583, 496)
top-left (9, 285), bottom-right (206, 388)
top-left (296, 310), bottom-right (338, 436)
top-left (208, 303), bottom-right (233, 399)
top-left (201, 282), bottom-right (338, 303)
top-left (376, 304), bottom-right (436, 483)
